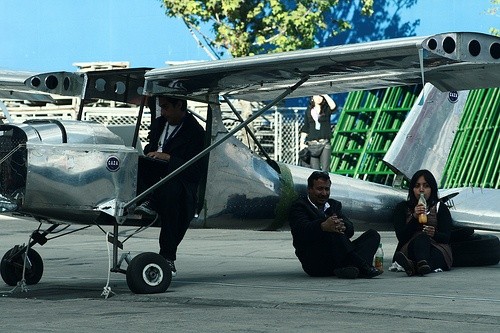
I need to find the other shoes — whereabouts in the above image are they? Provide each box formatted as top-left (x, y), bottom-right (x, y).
top-left (167, 259), bottom-right (177, 276)
top-left (359, 262), bottom-right (383, 279)
top-left (335, 266), bottom-right (359, 278)
top-left (416, 260), bottom-right (430, 275)
top-left (395, 251), bottom-right (416, 276)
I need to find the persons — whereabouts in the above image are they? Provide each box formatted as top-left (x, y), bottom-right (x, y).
top-left (288, 171), bottom-right (384, 279)
top-left (133, 96), bottom-right (206, 276)
top-left (299, 94), bottom-right (337, 171)
top-left (393, 169), bottom-right (455, 276)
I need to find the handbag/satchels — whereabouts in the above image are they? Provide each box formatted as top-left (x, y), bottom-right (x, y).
top-left (298, 147), bottom-right (310, 165)
top-left (309, 146), bottom-right (324, 157)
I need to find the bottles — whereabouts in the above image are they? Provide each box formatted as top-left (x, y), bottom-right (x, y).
top-left (375, 244), bottom-right (385, 271)
top-left (418, 192), bottom-right (427, 223)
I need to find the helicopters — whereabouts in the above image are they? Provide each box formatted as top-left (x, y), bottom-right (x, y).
top-left (0, 29), bottom-right (500, 300)
